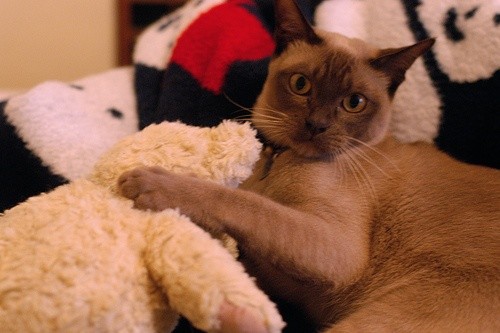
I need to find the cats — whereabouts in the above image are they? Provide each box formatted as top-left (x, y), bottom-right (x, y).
top-left (118, 0), bottom-right (500, 333)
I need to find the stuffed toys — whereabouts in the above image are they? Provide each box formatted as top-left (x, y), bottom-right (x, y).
top-left (0, 119), bottom-right (287, 333)
